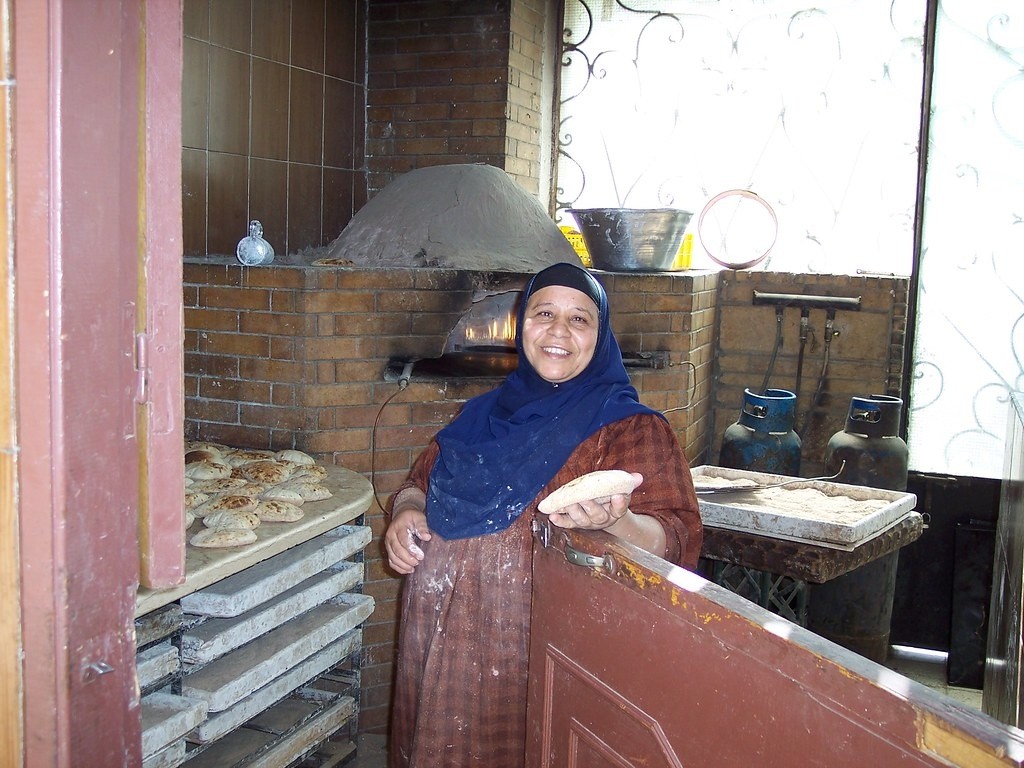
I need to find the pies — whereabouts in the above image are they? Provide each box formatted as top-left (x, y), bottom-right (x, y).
top-left (537, 467), bottom-right (635, 514)
top-left (184, 444), bottom-right (332, 547)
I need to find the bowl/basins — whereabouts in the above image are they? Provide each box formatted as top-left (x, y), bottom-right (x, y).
top-left (566, 209), bottom-right (693, 271)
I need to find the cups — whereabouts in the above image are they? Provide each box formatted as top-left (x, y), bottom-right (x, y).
top-left (236, 220), bottom-right (274, 265)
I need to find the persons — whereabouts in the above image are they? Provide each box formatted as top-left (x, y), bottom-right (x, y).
top-left (385, 262), bottom-right (703, 768)
